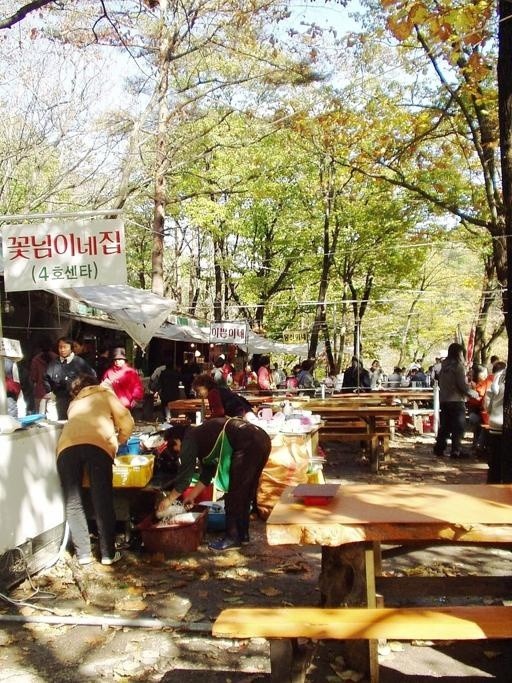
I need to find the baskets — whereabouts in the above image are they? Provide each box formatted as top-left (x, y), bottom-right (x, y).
top-left (199, 500), bottom-right (226, 532)
top-left (80, 454), bottom-right (156, 489)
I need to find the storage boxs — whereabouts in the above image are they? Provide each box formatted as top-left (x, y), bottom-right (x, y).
top-left (398, 409), bottom-right (441, 433)
top-left (178, 482), bottom-right (213, 504)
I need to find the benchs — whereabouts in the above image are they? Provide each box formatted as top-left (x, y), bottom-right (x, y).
top-left (319, 415), bottom-right (394, 470)
top-left (211, 605), bottom-right (511, 683)
top-left (407, 396), bottom-right (433, 409)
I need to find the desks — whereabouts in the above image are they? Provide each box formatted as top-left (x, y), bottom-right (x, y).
top-left (265, 483), bottom-right (512, 682)
top-left (304, 406), bottom-right (401, 416)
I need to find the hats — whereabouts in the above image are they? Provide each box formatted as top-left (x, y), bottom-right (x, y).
top-left (110, 347), bottom-right (129, 362)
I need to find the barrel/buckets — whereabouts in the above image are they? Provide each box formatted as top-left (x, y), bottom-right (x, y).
top-left (117, 438), bottom-right (141, 455)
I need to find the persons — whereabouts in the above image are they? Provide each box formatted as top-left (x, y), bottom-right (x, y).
top-left (156, 416), bottom-right (271, 551)
top-left (54, 376), bottom-right (135, 565)
top-left (28, 334), bottom-right (507, 462)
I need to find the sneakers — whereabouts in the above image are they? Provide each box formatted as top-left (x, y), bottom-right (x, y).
top-left (238, 530), bottom-right (250, 545)
top-left (208, 537), bottom-right (242, 552)
top-left (101, 551), bottom-right (125, 565)
top-left (432, 446), bottom-right (472, 459)
top-left (78, 551), bottom-right (100, 566)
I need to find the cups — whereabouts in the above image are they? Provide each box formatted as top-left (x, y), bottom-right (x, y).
top-left (258, 407), bottom-right (272, 419)
